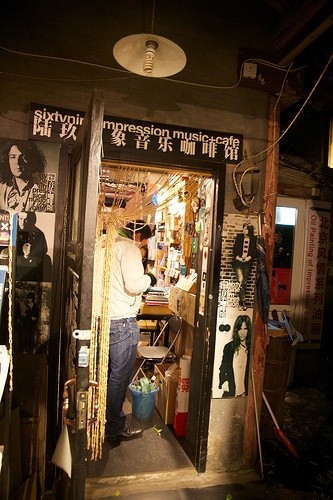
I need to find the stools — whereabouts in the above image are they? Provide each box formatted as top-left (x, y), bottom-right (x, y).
top-left (138, 322), bottom-right (157, 346)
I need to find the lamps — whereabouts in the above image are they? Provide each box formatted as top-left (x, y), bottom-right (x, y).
top-left (112, 4), bottom-right (188, 79)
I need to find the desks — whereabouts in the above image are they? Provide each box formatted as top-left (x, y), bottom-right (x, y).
top-left (138, 306), bottom-right (175, 348)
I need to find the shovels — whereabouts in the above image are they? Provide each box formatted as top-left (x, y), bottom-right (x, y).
top-left (263, 392), bottom-right (299, 459)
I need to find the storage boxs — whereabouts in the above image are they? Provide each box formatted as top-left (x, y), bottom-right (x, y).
top-left (154, 363), bottom-right (180, 425)
top-left (175, 277), bottom-right (197, 295)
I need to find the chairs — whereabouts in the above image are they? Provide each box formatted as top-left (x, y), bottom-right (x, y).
top-left (131, 315), bottom-right (183, 390)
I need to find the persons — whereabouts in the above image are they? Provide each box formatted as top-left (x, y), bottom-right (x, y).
top-left (16, 239), bottom-right (53, 282)
top-left (140, 246), bottom-right (148, 271)
top-left (93, 219), bottom-right (151, 441)
top-left (233, 222), bottom-right (257, 311)
top-left (16, 211), bottom-right (47, 254)
top-left (22, 291), bottom-right (41, 354)
top-left (0, 139), bottom-right (50, 212)
top-left (219, 315), bottom-right (252, 397)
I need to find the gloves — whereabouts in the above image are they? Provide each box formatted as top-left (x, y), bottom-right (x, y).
top-left (144, 272), bottom-right (157, 287)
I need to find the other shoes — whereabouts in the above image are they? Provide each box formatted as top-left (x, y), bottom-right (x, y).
top-left (240, 301), bottom-right (246, 311)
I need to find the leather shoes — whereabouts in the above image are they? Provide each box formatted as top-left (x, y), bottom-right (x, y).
top-left (108, 426), bottom-right (143, 440)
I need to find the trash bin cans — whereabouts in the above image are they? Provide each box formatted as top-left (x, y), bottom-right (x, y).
top-left (260, 320), bottom-right (296, 430)
top-left (128, 380), bottom-right (160, 420)
